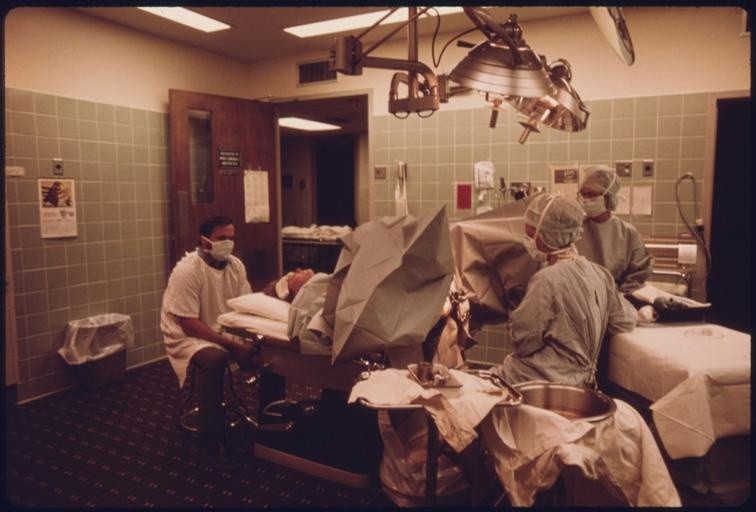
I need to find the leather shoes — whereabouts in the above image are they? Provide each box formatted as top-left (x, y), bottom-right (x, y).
top-left (260, 400), bottom-right (314, 422)
top-left (208, 441), bottom-right (240, 468)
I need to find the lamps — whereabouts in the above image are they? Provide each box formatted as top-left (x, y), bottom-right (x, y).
top-left (329, 6), bottom-right (634, 145)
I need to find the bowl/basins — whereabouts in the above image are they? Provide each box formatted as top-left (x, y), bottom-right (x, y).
top-left (507, 379), bottom-right (617, 424)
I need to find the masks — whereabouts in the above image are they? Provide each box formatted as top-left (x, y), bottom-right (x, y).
top-left (576, 193), bottom-right (610, 219)
top-left (524, 233), bottom-right (547, 264)
top-left (202, 236), bottom-right (234, 262)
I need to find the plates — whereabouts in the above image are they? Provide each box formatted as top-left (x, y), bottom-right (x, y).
top-left (406, 361), bottom-right (463, 388)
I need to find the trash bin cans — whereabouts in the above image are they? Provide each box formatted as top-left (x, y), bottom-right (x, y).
top-left (68, 313), bottom-right (131, 390)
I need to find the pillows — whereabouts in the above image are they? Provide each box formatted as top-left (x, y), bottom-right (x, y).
top-left (225, 292), bottom-right (290, 324)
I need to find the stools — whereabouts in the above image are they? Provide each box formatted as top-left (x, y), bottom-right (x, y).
top-left (180, 352), bottom-right (248, 435)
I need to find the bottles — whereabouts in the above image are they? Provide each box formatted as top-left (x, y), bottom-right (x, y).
top-left (415, 361), bottom-right (434, 382)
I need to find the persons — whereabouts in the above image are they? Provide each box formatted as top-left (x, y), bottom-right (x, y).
top-left (260, 263), bottom-right (316, 306)
top-left (156, 213), bottom-right (291, 475)
top-left (480, 189), bottom-right (640, 398)
top-left (568, 161), bottom-right (655, 392)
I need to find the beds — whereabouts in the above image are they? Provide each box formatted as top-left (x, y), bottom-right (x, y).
top-left (606, 313), bottom-right (752, 505)
top-left (216, 310), bottom-right (515, 490)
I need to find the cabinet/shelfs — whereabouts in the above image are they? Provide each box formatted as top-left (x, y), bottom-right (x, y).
top-left (353, 367), bottom-right (523, 510)
